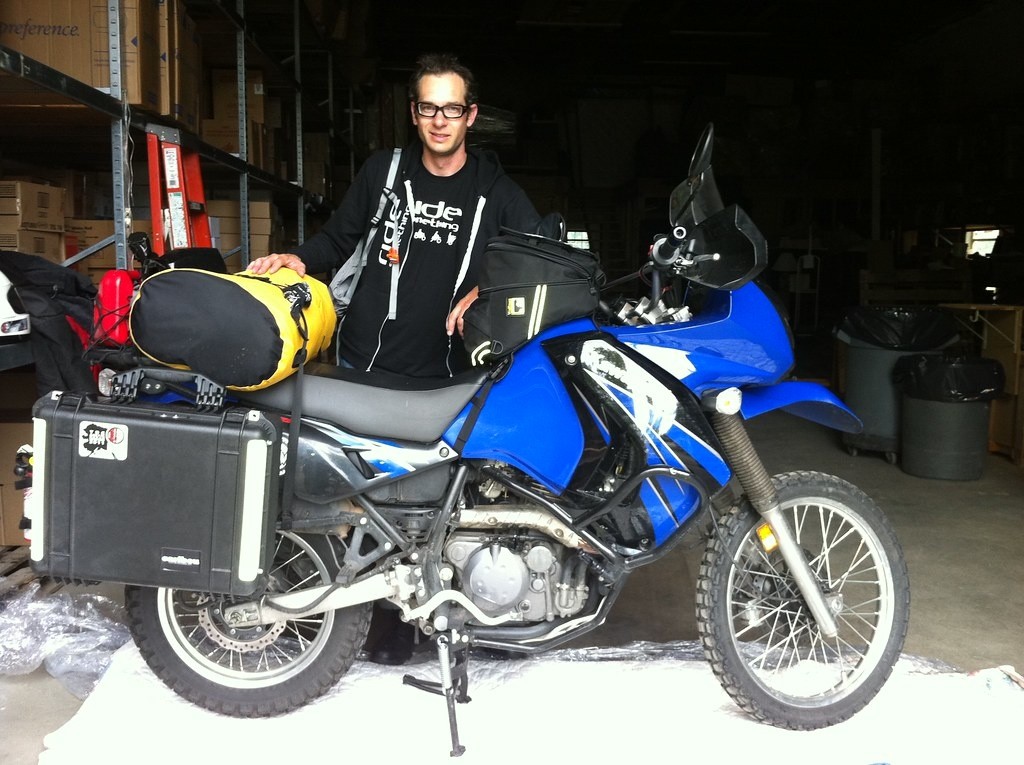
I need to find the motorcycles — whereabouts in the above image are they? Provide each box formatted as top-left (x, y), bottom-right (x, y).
top-left (12, 118), bottom-right (909, 764)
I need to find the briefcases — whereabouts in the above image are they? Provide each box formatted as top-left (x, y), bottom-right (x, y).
top-left (26, 365), bottom-right (283, 596)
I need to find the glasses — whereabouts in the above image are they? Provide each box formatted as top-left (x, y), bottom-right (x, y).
top-left (412, 100), bottom-right (470, 119)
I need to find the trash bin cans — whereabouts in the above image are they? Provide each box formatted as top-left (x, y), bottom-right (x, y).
top-left (832, 304), bottom-right (962, 466)
top-left (899, 354), bottom-right (1006, 481)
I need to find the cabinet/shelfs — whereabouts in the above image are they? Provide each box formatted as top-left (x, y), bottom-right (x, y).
top-left (937, 302), bottom-right (1024, 465)
top-left (1, 1), bottom-right (384, 376)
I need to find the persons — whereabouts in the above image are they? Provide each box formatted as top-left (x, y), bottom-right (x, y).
top-left (245, 52), bottom-right (548, 665)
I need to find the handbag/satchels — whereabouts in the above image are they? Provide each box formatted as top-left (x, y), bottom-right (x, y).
top-left (328, 240), bottom-right (367, 313)
top-left (466, 210), bottom-right (603, 359)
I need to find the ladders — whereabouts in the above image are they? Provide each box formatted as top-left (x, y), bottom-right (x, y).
top-left (143, 124), bottom-right (212, 257)
